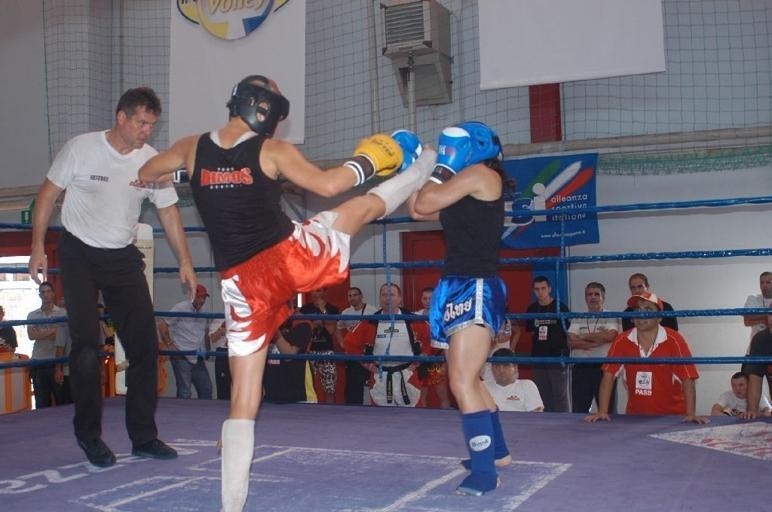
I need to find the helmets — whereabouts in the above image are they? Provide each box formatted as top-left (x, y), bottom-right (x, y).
top-left (226, 75), bottom-right (290, 139)
top-left (447, 119), bottom-right (503, 168)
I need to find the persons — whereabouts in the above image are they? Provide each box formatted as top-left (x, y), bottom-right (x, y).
top-left (743, 271), bottom-right (772, 356)
top-left (26, 85), bottom-right (201, 469)
top-left (710, 371), bottom-right (771, 419)
top-left (479, 273), bottom-right (711, 427)
top-left (2, 273), bottom-right (231, 425)
top-left (263, 283), bottom-right (460, 408)
top-left (391, 118), bottom-right (520, 500)
top-left (134, 72), bottom-right (441, 511)
top-left (736, 301), bottom-right (771, 423)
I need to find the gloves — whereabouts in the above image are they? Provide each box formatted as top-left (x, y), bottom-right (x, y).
top-left (388, 128), bottom-right (426, 175)
top-left (429, 127), bottom-right (476, 185)
top-left (343, 133), bottom-right (402, 187)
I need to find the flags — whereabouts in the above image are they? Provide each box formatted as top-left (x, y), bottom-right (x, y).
top-left (492, 148), bottom-right (601, 251)
top-left (167, 1), bottom-right (311, 150)
top-left (477, 1), bottom-right (668, 95)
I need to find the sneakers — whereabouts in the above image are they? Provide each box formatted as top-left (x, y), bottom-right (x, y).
top-left (129, 433), bottom-right (181, 463)
top-left (74, 432), bottom-right (116, 469)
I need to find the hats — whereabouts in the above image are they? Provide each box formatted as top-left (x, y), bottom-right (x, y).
top-left (197, 283), bottom-right (210, 298)
top-left (627, 291), bottom-right (665, 315)
